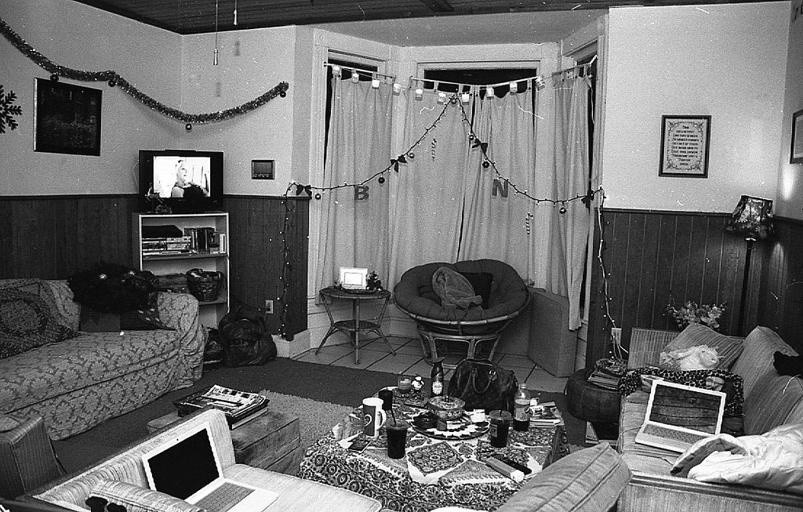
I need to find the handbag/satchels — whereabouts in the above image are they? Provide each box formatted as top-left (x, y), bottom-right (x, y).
top-left (218, 308), bottom-right (277, 365)
top-left (447, 358), bottom-right (517, 414)
top-left (69, 267), bottom-right (159, 312)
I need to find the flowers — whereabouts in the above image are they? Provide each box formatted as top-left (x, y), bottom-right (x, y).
top-left (663, 302), bottom-right (727, 329)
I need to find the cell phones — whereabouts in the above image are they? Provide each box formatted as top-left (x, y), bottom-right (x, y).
top-left (349, 440), bottom-right (371, 453)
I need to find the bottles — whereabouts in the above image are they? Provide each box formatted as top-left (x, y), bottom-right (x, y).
top-left (412, 376), bottom-right (424, 393)
top-left (184, 227), bottom-right (215, 253)
top-left (436, 410), bottom-right (448, 431)
top-left (430, 360), bottom-right (444, 398)
top-left (513, 382), bottom-right (530, 432)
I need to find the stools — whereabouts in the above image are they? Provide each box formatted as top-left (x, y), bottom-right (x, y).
top-left (145, 408), bottom-right (302, 475)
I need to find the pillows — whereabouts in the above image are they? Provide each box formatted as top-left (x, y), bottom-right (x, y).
top-left (459, 272), bottom-right (493, 309)
top-left (86, 479), bottom-right (199, 512)
top-left (80, 277), bottom-right (159, 331)
top-left (498, 441), bottom-right (633, 512)
top-left (663, 324), bottom-right (743, 370)
top-left (0, 281), bottom-right (82, 358)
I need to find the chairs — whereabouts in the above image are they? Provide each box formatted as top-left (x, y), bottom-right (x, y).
top-left (0, 413), bottom-right (62, 501)
top-left (394, 259), bottom-right (531, 369)
top-left (18, 408), bottom-right (382, 512)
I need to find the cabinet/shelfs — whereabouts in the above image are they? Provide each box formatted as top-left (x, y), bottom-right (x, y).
top-left (131, 212), bottom-right (230, 364)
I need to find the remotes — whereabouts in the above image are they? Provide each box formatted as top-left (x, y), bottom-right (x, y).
top-left (486, 456), bottom-right (524, 483)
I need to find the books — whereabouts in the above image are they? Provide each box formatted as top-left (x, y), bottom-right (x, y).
top-left (171, 385), bottom-right (271, 429)
top-left (142, 224), bottom-right (227, 257)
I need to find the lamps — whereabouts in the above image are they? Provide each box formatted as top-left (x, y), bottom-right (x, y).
top-left (415, 88), bottom-right (470, 103)
top-left (725, 194), bottom-right (775, 337)
top-left (486, 77), bottom-right (545, 98)
top-left (332, 66), bottom-right (401, 94)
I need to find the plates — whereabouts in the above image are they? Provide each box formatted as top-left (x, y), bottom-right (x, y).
top-left (413, 415), bottom-right (436, 427)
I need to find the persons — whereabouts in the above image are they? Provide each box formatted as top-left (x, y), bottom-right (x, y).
top-left (171, 160), bottom-right (209, 197)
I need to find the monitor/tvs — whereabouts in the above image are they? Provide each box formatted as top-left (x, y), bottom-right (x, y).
top-left (139, 149), bottom-right (224, 214)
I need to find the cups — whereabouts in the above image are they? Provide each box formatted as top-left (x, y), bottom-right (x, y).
top-left (385, 418), bottom-right (410, 460)
top-left (471, 408), bottom-right (486, 423)
top-left (378, 389), bottom-right (392, 411)
top-left (397, 374), bottom-right (411, 393)
top-left (489, 409), bottom-right (510, 448)
top-left (362, 397), bottom-right (386, 438)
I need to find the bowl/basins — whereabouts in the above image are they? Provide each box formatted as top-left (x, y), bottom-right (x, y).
top-left (427, 395), bottom-right (466, 420)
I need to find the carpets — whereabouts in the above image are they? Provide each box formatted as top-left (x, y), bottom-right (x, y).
top-left (56, 357), bottom-right (597, 470)
top-left (259, 389), bottom-right (584, 453)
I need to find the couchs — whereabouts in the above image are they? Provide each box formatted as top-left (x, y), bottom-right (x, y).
top-left (618, 326), bottom-right (803, 511)
top-left (0, 279), bottom-right (204, 440)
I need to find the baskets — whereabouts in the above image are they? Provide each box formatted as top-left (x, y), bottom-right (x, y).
top-left (186, 267), bottom-right (224, 301)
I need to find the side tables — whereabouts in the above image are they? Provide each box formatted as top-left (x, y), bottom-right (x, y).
top-left (315, 288), bottom-right (396, 365)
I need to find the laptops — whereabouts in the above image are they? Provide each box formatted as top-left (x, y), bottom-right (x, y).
top-left (141, 422), bottom-right (279, 512)
top-left (635, 380), bottom-right (727, 454)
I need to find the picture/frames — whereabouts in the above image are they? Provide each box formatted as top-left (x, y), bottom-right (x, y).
top-left (33, 77), bottom-right (104, 159)
top-left (790, 110), bottom-right (803, 164)
top-left (659, 115), bottom-right (711, 178)
